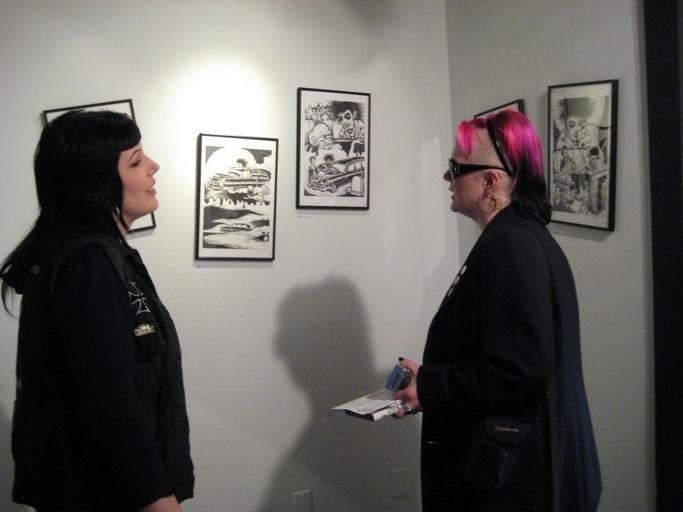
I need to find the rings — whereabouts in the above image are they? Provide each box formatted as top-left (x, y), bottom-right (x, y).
top-left (401, 404), bottom-right (416, 416)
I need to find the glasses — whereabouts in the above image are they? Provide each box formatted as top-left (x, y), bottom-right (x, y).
top-left (448, 158), bottom-right (512, 180)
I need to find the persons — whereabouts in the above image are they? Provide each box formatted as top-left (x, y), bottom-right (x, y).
top-left (390, 107), bottom-right (602, 512)
top-left (0, 110), bottom-right (196, 512)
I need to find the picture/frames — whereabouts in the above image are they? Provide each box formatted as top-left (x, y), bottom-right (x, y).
top-left (473, 79), bottom-right (617, 232)
